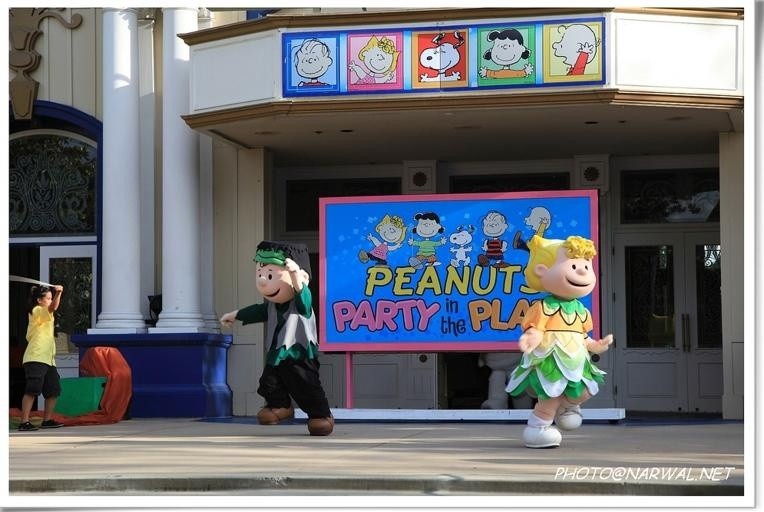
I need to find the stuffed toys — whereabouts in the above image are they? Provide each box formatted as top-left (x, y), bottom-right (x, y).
top-left (222, 240), bottom-right (334, 437)
top-left (504, 234), bottom-right (616, 446)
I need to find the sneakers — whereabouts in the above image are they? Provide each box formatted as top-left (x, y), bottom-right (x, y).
top-left (41, 419), bottom-right (65, 428)
top-left (18, 421), bottom-right (39, 432)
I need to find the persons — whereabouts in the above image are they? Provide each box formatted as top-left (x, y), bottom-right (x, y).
top-left (17, 284), bottom-right (63, 431)
